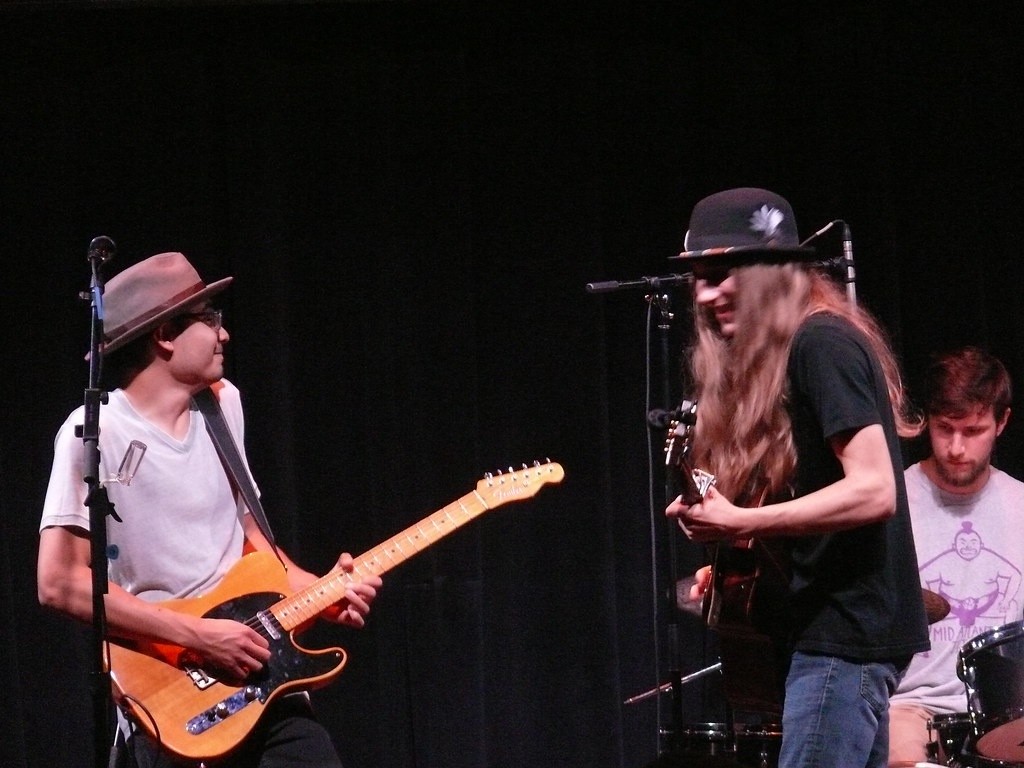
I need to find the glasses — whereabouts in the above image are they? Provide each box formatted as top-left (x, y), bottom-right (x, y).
top-left (182, 309), bottom-right (221, 329)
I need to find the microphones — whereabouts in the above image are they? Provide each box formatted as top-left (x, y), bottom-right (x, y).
top-left (88, 235), bottom-right (115, 265)
top-left (844, 224), bottom-right (856, 304)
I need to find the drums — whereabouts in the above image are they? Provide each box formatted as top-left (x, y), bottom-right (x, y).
top-left (927, 712), bottom-right (1024, 768)
top-left (656, 722), bottom-right (785, 768)
top-left (955, 620), bottom-right (1024, 764)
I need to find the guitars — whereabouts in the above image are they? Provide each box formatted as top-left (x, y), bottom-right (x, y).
top-left (663, 397), bottom-right (800, 693)
top-left (101, 457), bottom-right (567, 764)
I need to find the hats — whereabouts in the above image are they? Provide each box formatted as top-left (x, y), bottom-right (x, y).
top-left (84, 252), bottom-right (233, 362)
top-left (667, 188), bottom-right (815, 259)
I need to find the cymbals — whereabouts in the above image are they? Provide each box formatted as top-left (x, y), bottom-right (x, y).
top-left (922, 587), bottom-right (953, 626)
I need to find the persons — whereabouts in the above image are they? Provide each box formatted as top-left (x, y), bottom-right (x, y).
top-left (665, 189), bottom-right (932, 768)
top-left (37, 252), bottom-right (382, 768)
top-left (889, 350), bottom-right (1024, 768)
